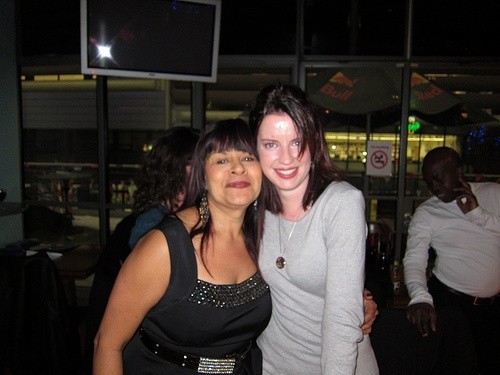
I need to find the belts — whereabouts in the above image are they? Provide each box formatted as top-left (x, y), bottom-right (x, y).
top-left (136, 323), bottom-right (256, 375)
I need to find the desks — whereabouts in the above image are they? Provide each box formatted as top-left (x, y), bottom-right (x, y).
top-left (0, 244), bottom-right (107, 280)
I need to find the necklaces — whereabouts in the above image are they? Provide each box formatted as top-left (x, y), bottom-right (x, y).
top-left (275, 204), bottom-right (304, 269)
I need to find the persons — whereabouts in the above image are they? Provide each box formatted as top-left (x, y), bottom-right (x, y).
top-left (92, 126), bottom-right (202, 343)
top-left (92, 117), bottom-right (380, 375)
top-left (401, 147), bottom-right (500, 375)
top-left (254, 85), bottom-right (380, 375)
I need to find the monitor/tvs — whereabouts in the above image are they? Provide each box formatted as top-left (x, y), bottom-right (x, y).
top-left (80, 0), bottom-right (221, 83)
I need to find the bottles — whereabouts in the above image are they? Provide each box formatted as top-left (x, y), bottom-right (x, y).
top-left (391, 256), bottom-right (400, 296)
top-left (378, 253), bottom-right (389, 297)
top-left (366, 233), bottom-right (378, 271)
